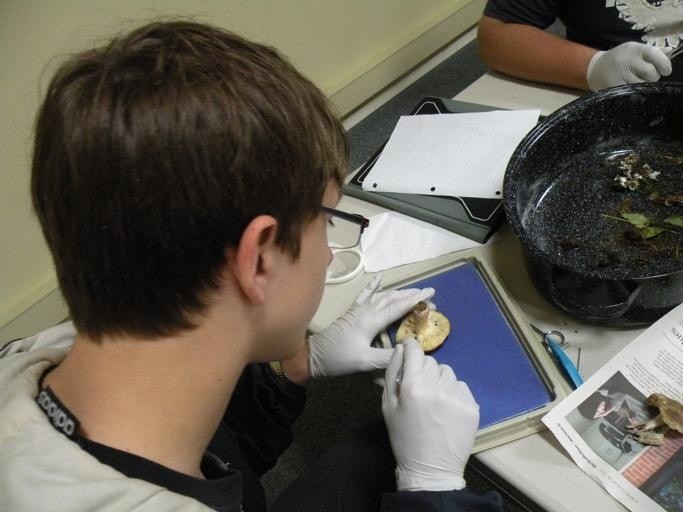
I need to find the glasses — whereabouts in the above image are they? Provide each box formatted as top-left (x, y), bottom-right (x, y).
top-left (312, 202), bottom-right (370, 250)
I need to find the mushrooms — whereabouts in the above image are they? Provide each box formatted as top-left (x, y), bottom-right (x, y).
top-left (624, 393), bottom-right (683, 446)
top-left (395, 300), bottom-right (451, 352)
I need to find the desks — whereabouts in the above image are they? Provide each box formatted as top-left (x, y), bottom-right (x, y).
top-left (306, 18), bottom-right (682, 512)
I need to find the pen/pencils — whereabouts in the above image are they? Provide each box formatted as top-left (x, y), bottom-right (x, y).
top-left (546, 334), bottom-right (584, 388)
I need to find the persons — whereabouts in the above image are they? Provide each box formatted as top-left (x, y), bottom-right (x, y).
top-left (0, 16), bottom-right (483, 512)
top-left (577, 370), bottom-right (628, 421)
top-left (464, 0), bottom-right (683, 94)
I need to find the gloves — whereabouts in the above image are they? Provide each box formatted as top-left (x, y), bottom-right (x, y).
top-left (379, 338), bottom-right (482, 491)
top-left (306, 274), bottom-right (436, 378)
top-left (585, 42), bottom-right (675, 94)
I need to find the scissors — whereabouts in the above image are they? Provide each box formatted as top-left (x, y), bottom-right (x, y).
top-left (529, 322), bottom-right (565, 353)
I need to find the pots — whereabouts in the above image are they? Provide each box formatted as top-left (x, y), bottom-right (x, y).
top-left (500, 81), bottom-right (683, 329)
